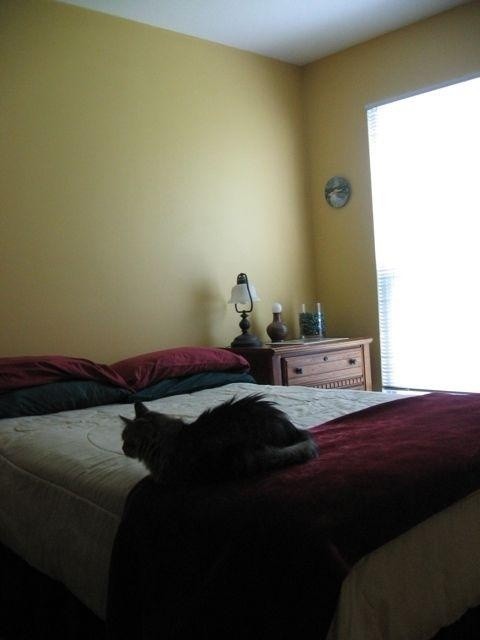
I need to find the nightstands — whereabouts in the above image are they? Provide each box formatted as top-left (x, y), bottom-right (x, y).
top-left (218, 337), bottom-right (374, 392)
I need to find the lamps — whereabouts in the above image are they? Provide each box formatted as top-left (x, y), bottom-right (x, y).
top-left (230, 273), bottom-right (263, 348)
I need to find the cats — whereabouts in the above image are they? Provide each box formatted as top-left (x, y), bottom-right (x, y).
top-left (117, 390), bottom-right (320, 491)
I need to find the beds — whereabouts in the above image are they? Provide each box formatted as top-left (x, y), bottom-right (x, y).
top-left (1, 372), bottom-right (480, 637)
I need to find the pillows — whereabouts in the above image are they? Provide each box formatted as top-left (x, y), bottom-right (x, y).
top-left (0, 346), bottom-right (251, 395)
